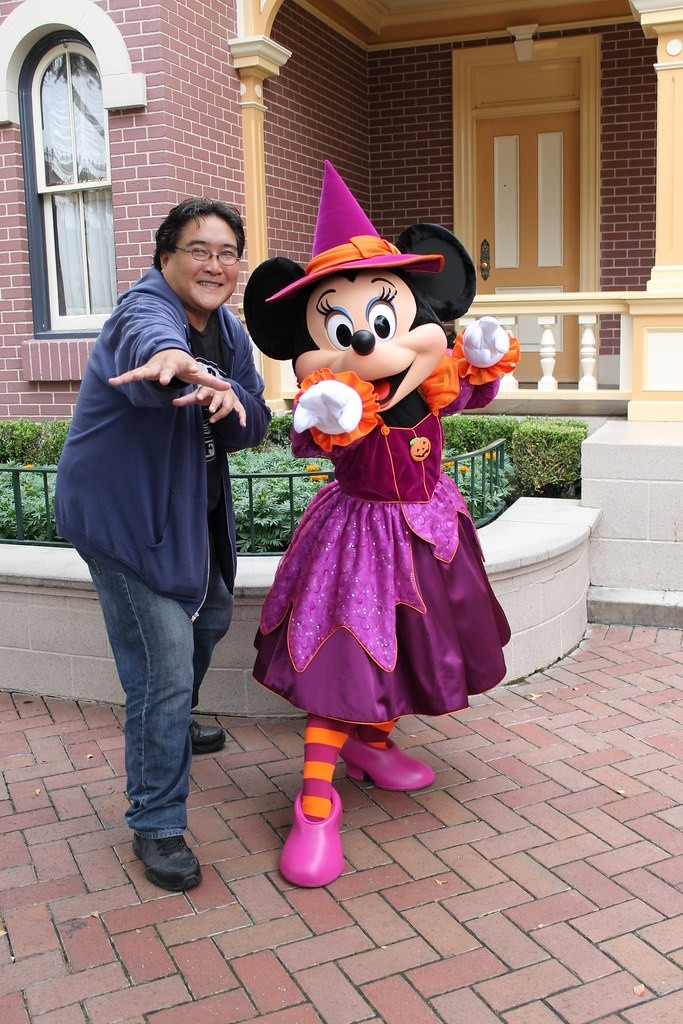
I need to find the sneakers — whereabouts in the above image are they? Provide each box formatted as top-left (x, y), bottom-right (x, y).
top-left (187, 718), bottom-right (226, 753)
top-left (132, 829), bottom-right (202, 892)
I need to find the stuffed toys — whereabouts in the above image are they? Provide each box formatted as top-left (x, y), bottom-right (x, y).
top-left (243, 162), bottom-right (522, 886)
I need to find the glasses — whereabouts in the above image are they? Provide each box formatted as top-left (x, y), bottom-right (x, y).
top-left (172, 247), bottom-right (239, 266)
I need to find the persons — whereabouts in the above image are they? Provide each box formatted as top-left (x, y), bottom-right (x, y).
top-left (54, 198), bottom-right (273, 890)
